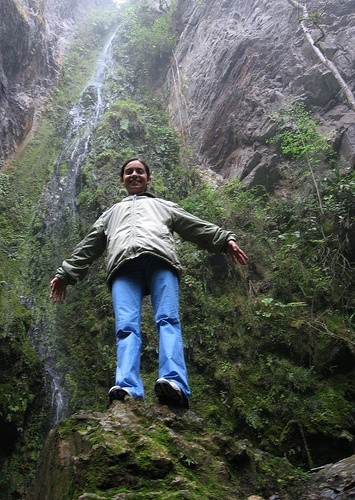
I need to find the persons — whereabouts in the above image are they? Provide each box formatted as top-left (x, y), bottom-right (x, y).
top-left (47, 158), bottom-right (250, 410)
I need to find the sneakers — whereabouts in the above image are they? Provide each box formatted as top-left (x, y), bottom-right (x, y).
top-left (154, 378), bottom-right (190, 410)
top-left (107, 386), bottom-right (134, 406)
